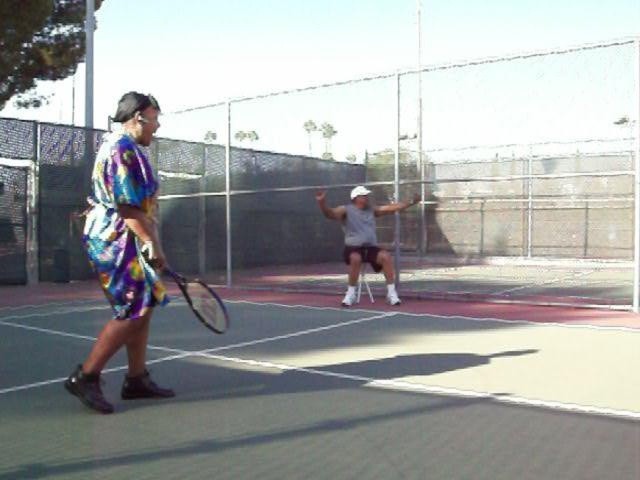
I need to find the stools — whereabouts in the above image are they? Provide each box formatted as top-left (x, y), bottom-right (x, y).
top-left (355, 253), bottom-right (377, 304)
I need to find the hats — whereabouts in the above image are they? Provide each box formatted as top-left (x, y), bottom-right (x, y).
top-left (350, 185), bottom-right (372, 201)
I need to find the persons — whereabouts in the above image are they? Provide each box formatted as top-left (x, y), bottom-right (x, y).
top-left (63, 91), bottom-right (176, 415)
top-left (315, 185), bottom-right (422, 307)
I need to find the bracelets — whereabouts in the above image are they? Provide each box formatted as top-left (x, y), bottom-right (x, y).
top-left (408, 196), bottom-right (414, 207)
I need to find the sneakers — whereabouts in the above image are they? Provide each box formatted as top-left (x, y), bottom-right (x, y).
top-left (120, 369), bottom-right (175, 400)
top-left (64, 365), bottom-right (114, 413)
top-left (341, 290), bottom-right (357, 306)
top-left (384, 289), bottom-right (402, 306)
